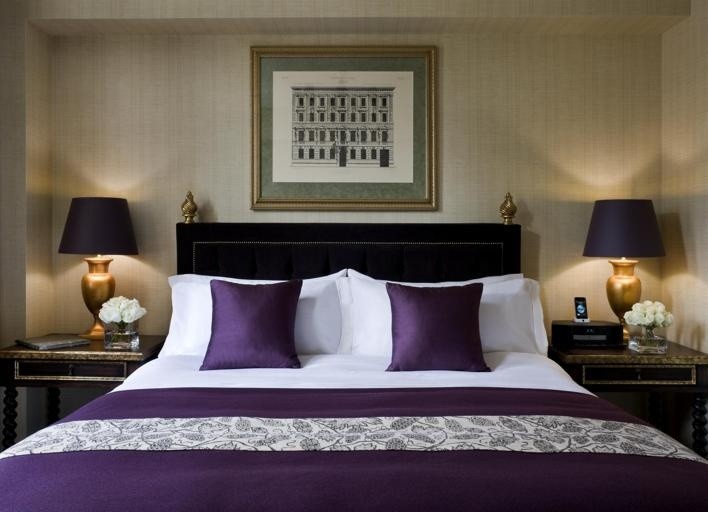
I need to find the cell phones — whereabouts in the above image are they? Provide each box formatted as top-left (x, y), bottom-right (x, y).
top-left (575, 297), bottom-right (587, 319)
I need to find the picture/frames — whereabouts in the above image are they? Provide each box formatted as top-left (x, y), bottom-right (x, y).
top-left (250, 44), bottom-right (439, 212)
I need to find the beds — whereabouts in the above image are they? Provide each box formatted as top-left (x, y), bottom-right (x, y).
top-left (1, 191), bottom-right (706, 511)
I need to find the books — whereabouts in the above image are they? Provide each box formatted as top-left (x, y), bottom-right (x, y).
top-left (14, 330), bottom-right (91, 350)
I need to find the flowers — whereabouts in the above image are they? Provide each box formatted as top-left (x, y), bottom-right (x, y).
top-left (623, 299), bottom-right (673, 327)
top-left (97, 295), bottom-right (148, 344)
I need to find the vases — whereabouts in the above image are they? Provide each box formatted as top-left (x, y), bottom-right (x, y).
top-left (628, 327), bottom-right (667, 355)
top-left (104, 323), bottom-right (140, 351)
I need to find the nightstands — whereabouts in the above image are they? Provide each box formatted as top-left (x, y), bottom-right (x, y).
top-left (547, 336), bottom-right (707, 456)
top-left (2, 332), bottom-right (167, 448)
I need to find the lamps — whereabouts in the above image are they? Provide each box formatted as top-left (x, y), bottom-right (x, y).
top-left (583, 199), bottom-right (665, 340)
top-left (58, 197), bottom-right (139, 341)
